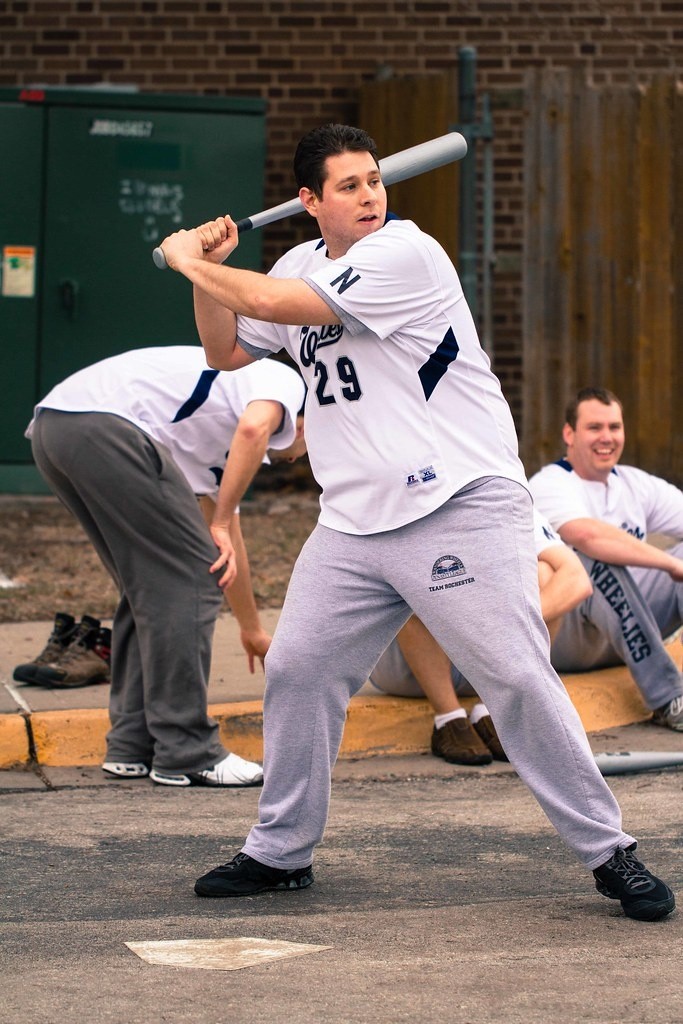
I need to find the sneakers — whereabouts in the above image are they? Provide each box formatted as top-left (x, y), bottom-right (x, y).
top-left (194, 852), bottom-right (315, 898)
top-left (12, 613), bottom-right (81, 687)
top-left (101, 762), bottom-right (153, 777)
top-left (149, 751), bottom-right (263, 786)
top-left (593, 844), bottom-right (676, 922)
top-left (36, 615), bottom-right (112, 686)
top-left (652, 695), bottom-right (683, 731)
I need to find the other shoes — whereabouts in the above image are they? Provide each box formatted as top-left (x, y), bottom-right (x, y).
top-left (473, 715), bottom-right (510, 762)
top-left (431, 717), bottom-right (493, 766)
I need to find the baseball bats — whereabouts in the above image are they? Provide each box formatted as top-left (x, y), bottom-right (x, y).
top-left (151, 130), bottom-right (470, 273)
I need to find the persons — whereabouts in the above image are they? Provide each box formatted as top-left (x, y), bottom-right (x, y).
top-left (369, 505), bottom-right (593, 766)
top-left (23, 344), bottom-right (308, 789)
top-left (527, 385), bottom-right (682, 731)
top-left (159, 128), bottom-right (676, 923)
